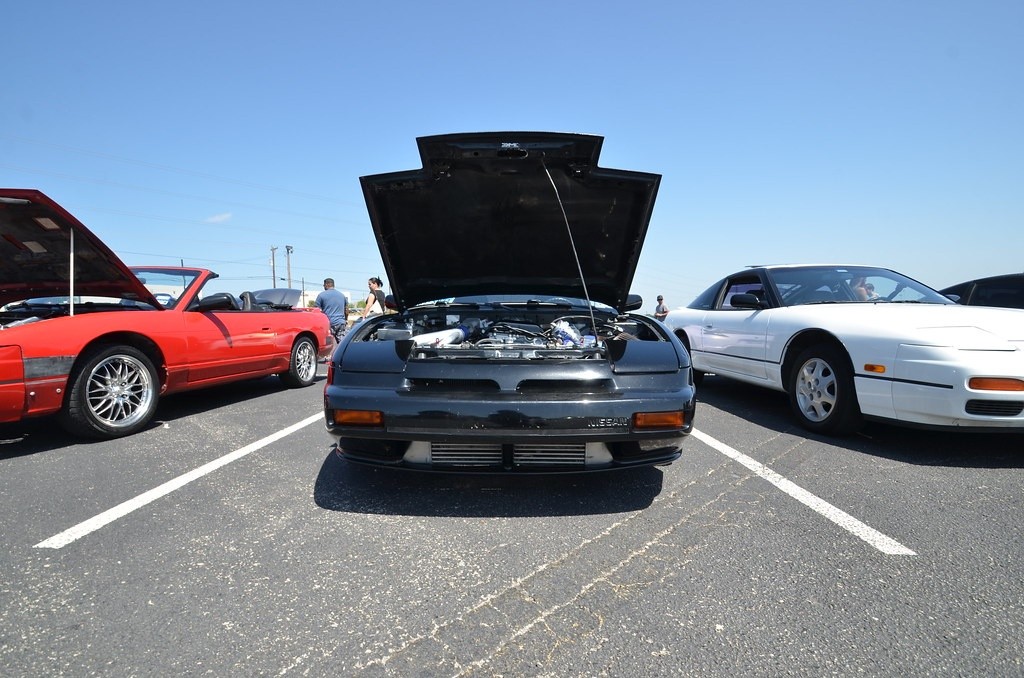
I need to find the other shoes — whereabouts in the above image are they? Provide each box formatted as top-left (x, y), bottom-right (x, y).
top-left (323, 360), bottom-right (330, 364)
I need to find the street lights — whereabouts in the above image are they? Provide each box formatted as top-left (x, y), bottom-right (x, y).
top-left (270, 244), bottom-right (278, 288)
top-left (284, 245), bottom-right (294, 288)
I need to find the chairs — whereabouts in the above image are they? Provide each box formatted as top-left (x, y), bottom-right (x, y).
top-left (212, 293), bottom-right (239, 310)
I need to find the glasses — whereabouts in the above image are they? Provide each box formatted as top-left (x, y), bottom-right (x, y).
top-left (657, 299), bottom-right (663, 301)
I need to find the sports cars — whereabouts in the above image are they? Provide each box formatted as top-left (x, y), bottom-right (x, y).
top-left (325, 128), bottom-right (695, 478)
top-left (660, 266), bottom-right (1024, 437)
top-left (0, 186), bottom-right (337, 441)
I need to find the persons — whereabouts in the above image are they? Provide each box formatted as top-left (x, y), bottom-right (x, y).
top-left (363, 276), bottom-right (385, 318)
top-left (313, 278), bottom-right (350, 345)
top-left (848, 275), bottom-right (881, 301)
top-left (653, 295), bottom-right (668, 321)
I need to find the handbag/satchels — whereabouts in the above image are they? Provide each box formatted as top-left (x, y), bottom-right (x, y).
top-left (366, 290), bottom-right (384, 318)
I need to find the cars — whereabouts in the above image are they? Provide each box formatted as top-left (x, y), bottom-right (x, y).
top-left (917, 272), bottom-right (1024, 308)
